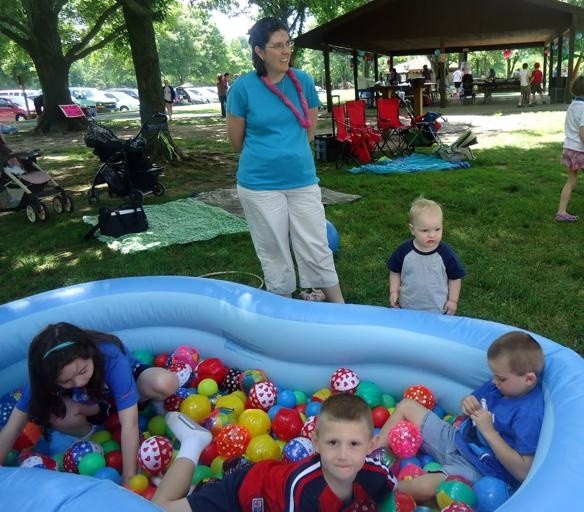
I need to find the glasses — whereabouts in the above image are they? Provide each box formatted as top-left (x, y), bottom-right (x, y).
top-left (262, 39), bottom-right (295, 49)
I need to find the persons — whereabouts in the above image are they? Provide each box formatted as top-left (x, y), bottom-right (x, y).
top-left (387, 63), bottom-right (569, 108)
top-left (217, 75), bottom-right (228, 117)
top-left (556, 74), bottom-right (584, 221)
top-left (226, 16), bottom-right (345, 305)
top-left (0, 321), bottom-right (179, 485)
top-left (152, 392), bottom-right (397, 512)
top-left (369, 329), bottom-right (544, 502)
top-left (385, 196), bottom-right (467, 316)
top-left (162, 80), bottom-right (176, 123)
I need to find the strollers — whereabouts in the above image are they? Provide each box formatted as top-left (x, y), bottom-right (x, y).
top-left (84, 111), bottom-right (170, 206)
top-left (0, 130), bottom-right (74, 223)
top-left (394, 89), bottom-right (449, 158)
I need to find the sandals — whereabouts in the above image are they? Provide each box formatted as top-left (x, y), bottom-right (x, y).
top-left (555, 212), bottom-right (579, 222)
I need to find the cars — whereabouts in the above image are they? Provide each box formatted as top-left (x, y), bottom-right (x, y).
top-left (172, 83), bottom-right (221, 105)
top-left (0, 86), bottom-right (141, 123)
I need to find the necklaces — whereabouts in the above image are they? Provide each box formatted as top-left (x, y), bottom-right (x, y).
top-left (260, 69), bottom-right (311, 129)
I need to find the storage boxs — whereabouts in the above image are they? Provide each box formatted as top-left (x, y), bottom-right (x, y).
top-left (313, 132), bottom-right (340, 162)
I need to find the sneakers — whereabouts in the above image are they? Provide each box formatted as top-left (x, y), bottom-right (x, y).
top-left (517, 101), bottom-right (547, 108)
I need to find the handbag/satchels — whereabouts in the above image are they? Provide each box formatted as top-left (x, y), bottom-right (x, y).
top-left (434, 130), bottom-right (478, 163)
top-left (99, 200), bottom-right (148, 237)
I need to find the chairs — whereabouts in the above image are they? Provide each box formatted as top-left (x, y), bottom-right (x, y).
top-left (373, 94), bottom-right (417, 158)
top-left (329, 103), bottom-right (373, 170)
top-left (343, 99), bottom-right (387, 158)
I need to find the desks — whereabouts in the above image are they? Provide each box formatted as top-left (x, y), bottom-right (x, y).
top-left (378, 83), bottom-right (438, 104)
